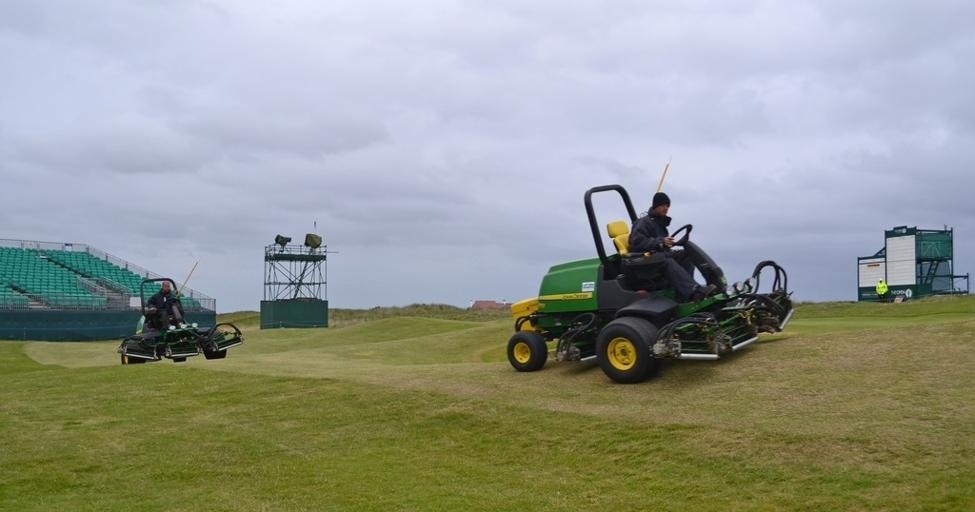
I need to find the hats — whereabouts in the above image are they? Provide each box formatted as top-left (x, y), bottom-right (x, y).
top-left (651, 192), bottom-right (671, 208)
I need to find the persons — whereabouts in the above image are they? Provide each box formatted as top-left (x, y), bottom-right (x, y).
top-left (876, 279), bottom-right (889, 302)
top-left (143, 279), bottom-right (184, 331)
top-left (627, 193), bottom-right (718, 303)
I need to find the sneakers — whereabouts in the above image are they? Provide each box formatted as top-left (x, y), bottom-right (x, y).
top-left (694, 283), bottom-right (717, 300)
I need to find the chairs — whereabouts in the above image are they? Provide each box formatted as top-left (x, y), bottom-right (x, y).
top-left (606, 219), bottom-right (648, 261)
top-left (2, 248), bottom-right (205, 311)
